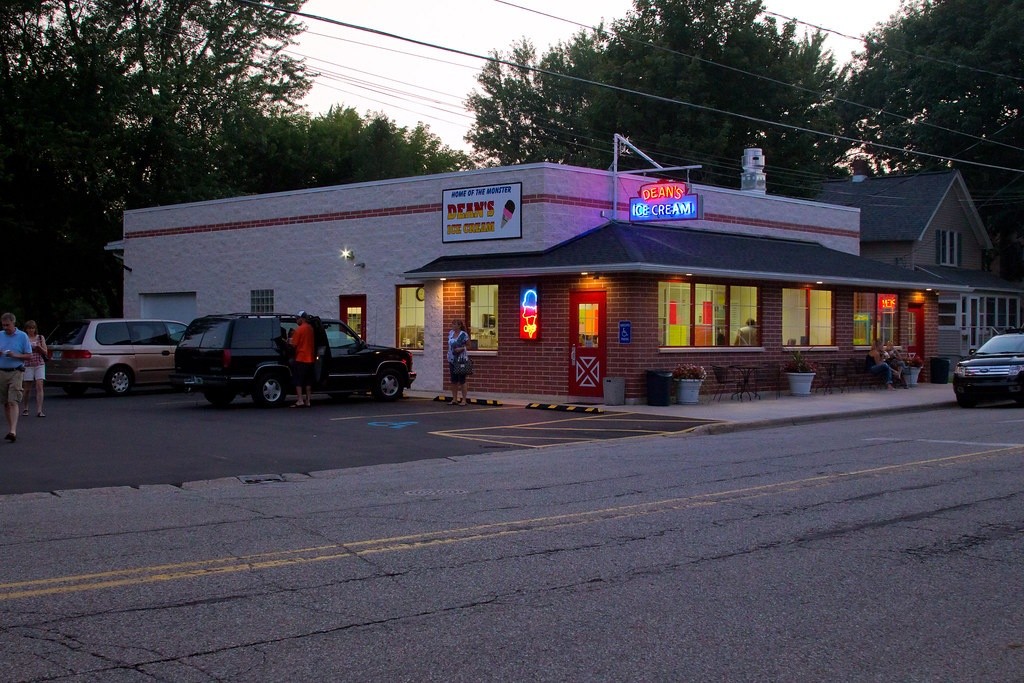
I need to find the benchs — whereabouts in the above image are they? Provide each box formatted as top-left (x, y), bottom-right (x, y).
top-left (840, 358), bottom-right (912, 393)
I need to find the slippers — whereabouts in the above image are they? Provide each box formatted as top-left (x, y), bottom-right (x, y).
top-left (304, 402), bottom-right (310, 407)
top-left (291, 403), bottom-right (304, 407)
top-left (23, 409), bottom-right (28, 416)
top-left (459, 402), bottom-right (467, 406)
top-left (37, 412), bottom-right (46, 417)
top-left (448, 401), bottom-right (458, 405)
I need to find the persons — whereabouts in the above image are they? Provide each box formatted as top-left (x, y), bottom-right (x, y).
top-left (866, 338), bottom-right (908, 389)
top-left (287, 310), bottom-right (314, 408)
top-left (21, 320), bottom-right (47, 416)
top-left (447, 319), bottom-right (472, 406)
top-left (0, 313), bottom-right (32, 442)
top-left (735, 318), bottom-right (756, 345)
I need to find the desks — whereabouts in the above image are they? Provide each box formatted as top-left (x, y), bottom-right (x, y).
top-left (814, 361), bottom-right (844, 395)
top-left (730, 364), bottom-right (763, 400)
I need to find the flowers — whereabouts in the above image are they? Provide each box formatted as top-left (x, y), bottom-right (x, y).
top-left (902, 357), bottom-right (924, 368)
top-left (676, 363), bottom-right (708, 379)
top-left (780, 347), bottom-right (816, 373)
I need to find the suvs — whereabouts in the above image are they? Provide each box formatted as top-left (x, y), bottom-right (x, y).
top-left (174, 311), bottom-right (418, 409)
top-left (46, 317), bottom-right (190, 398)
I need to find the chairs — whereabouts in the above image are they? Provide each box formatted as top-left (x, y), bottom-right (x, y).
top-left (710, 364), bottom-right (740, 402)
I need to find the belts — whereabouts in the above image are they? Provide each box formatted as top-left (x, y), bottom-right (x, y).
top-left (0, 367), bottom-right (18, 372)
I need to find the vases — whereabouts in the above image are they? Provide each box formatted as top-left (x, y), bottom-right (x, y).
top-left (903, 367), bottom-right (922, 385)
top-left (787, 372), bottom-right (817, 394)
top-left (674, 380), bottom-right (704, 404)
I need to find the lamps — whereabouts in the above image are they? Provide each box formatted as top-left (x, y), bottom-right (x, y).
top-left (347, 251), bottom-right (366, 268)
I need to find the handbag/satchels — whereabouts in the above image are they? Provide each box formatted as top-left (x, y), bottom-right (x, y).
top-left (39, 335), bottom-right (49, 362)
top-left (449, 351), bottom-right (473, 376)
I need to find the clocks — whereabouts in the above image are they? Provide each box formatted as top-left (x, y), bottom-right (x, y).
top-left (416, 288), bottom-right (425, 301)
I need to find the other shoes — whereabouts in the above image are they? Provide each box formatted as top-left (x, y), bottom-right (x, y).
top-left (4, 432), bottom-right (16, 441)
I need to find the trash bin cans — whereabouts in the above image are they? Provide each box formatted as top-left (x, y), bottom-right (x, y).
top-left (603, 377), bottom-right (625, 406)
top-left (646, 369), bottom-right (673, 405)
top-left (930, 357), bottom-right (949, 384)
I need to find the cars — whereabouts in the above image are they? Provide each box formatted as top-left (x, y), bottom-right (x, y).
top-left (952, 327), bottom-right (1024, 408)
